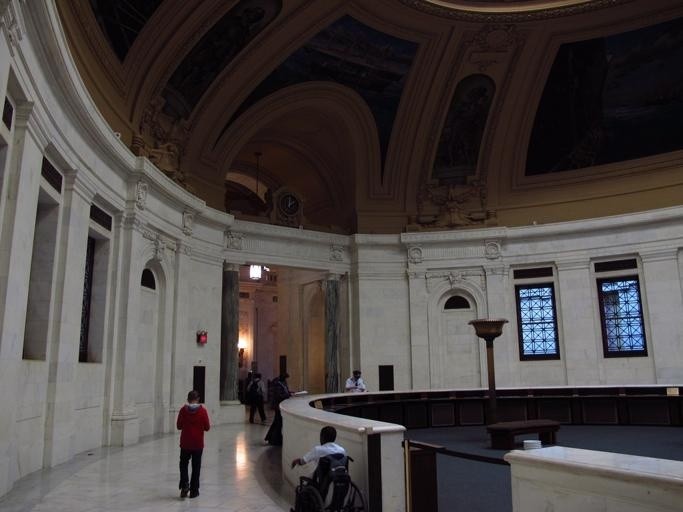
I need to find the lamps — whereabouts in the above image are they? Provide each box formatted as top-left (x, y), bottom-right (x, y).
top-left (249, 265), bottom-right (262, 281)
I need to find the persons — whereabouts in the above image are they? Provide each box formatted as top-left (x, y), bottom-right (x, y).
top-left (289, 426), bottom-right (347, 512)
top-left (246, 373), bottom-right (267, 424)
top-left (345, 369), bottom-right (367, 392)
top-left (177, 390), bottom-right (210, 498)
top-left (264, 373), bottom-right (295, 446)
top-left (239, 362), bottom-right (248, 404)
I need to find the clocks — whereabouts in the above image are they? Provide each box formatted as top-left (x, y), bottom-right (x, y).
top-left (279, 192), bottom-right (302, 217)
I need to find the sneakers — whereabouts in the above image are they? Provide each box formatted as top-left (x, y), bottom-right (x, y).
top-left (179, 484), bottom-right (200, 499)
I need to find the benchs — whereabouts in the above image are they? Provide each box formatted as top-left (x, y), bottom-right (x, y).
top-left (486, 419), bottom-right (560, 450)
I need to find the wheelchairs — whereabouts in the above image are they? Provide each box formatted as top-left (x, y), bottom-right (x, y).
top-left (290, 451), bottom-right (365, 511)
